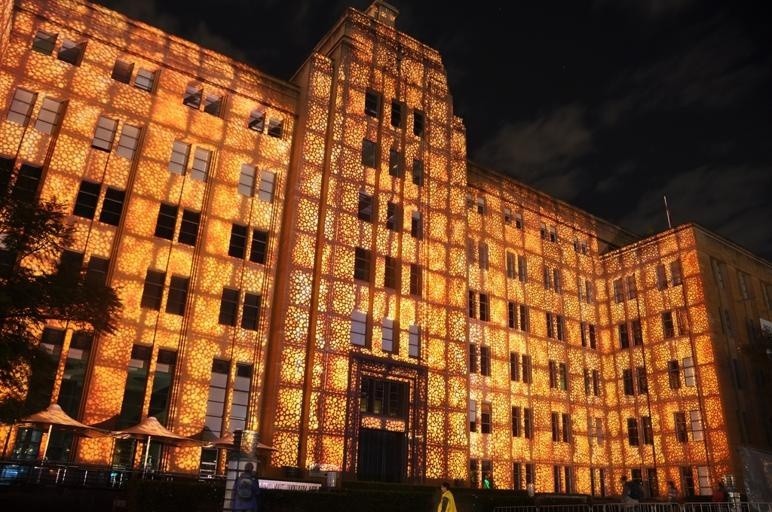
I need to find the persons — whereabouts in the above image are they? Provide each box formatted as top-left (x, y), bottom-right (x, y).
top-left (231, 462), bottom-right (260, 511)
top-left (619, 476), bottom-right (644, 501)
top-left (718, 478), bottom-right (725, 489)
top-left (437, 482), bottom-right (457, 511)
top-left (483, 474), bottom-right (489, 488)
top-left (666, 480), bottom-right (679, 501)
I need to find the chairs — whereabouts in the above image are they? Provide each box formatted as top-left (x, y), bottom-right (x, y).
top-left (0, 455), bottom-right (225, 500)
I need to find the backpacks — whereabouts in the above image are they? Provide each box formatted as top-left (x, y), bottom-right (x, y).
top-left (629, 480), bottom-right (644, 500)
top-left (236, 476), bottom-right (253, 501)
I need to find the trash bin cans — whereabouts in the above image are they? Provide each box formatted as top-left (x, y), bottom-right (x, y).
top-left (527, 483), bottom-right (535, 497)
top-left (728, 492), bottom-right (741, 512)
top-left (327, 472), bottom-right (337, 487)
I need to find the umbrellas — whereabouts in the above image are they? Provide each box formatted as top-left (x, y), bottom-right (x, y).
top-left (111, 416), bottom-right (196, 477)
top-left (14, 398), bottom-right (111, 479)
top-left (202, 430), bottom-right (278, 468)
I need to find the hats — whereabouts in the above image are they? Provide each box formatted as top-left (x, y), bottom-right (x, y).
top-left (620, 476), bottom-right (628, 481)
